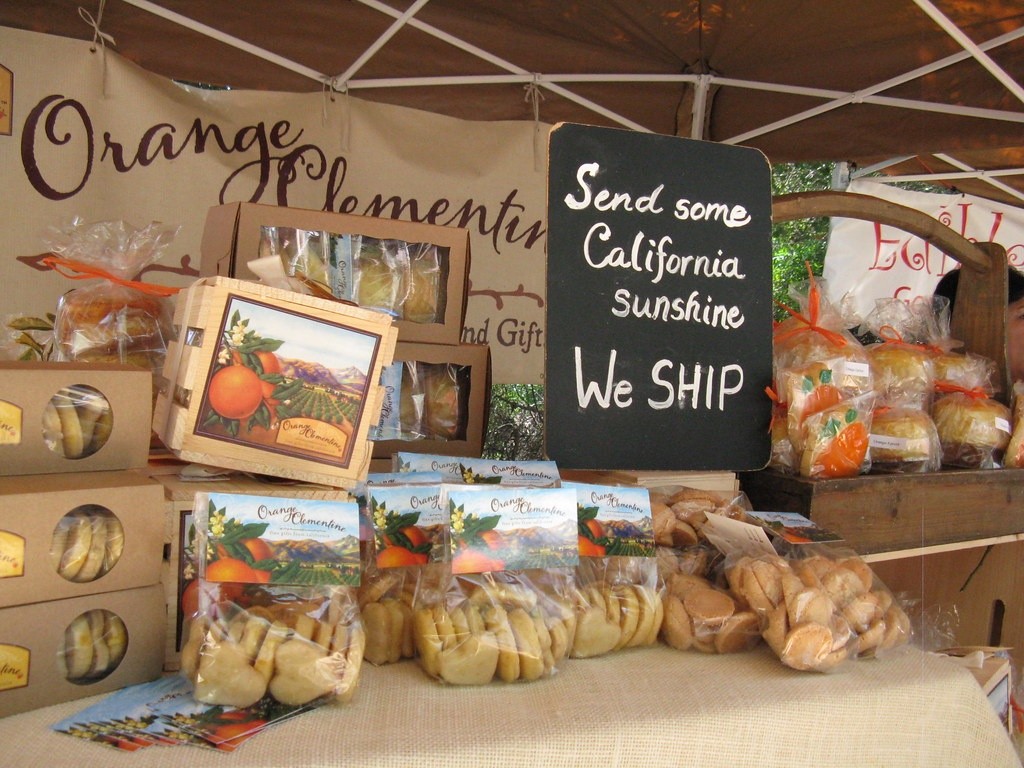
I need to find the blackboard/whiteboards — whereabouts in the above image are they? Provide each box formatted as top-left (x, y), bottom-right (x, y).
top-left (543, 121), bottom-right (774, 471)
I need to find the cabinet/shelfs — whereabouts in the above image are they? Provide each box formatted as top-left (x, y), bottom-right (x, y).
top-left (745, 467), bottom-right (1024, 679)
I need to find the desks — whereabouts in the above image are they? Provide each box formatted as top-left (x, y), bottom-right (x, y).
top-left (0, 659), bottom-right (1017, 768)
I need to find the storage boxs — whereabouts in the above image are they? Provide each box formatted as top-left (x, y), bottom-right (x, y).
top-left (371, 342), bottom-right (493, 460)
top-left (152, 276), bottom-right (398, 489)
top-left (0, 360), bottom-right (151, 477)
top-left (199, 200), bottom-right (471, 345)
top-left (0, 470), bottom-right (167, 720)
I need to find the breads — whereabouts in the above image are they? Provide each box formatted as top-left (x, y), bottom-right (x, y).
top-left (764, 318), bottom-right (1024, 476)
top-left (55, 288), bottom-right (174, 447)
top-left (177, 488), bottom-right (913, 707)
top-left (255, 236), bottom-right (463, 440)
top-left (42, 384), bottom-right (127, 678)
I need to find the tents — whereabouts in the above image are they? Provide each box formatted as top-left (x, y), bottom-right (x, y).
top-left (0, 0), bottom-right (1024, 239)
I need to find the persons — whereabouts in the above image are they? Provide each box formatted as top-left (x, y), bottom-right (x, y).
top-left (933, 265), bottom-right (1024, 382)
